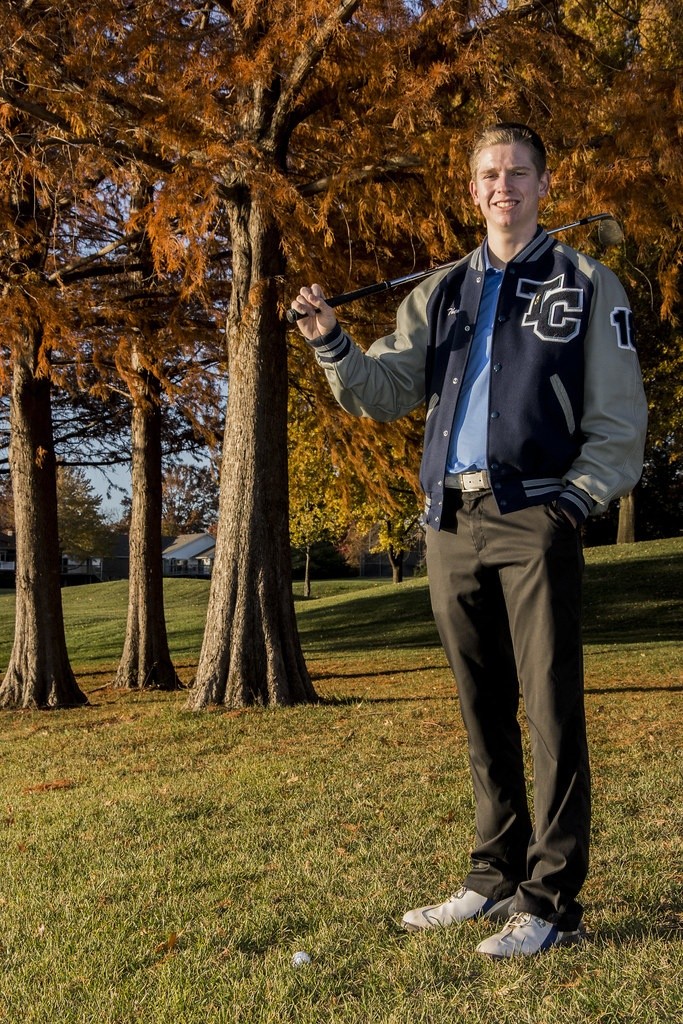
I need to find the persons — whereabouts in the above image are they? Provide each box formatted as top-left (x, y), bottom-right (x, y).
top-left (290, 123), bottom-right (648, 963)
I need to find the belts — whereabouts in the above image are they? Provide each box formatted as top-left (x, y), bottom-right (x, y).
top-left (444, 470), bottom-right (491, 491)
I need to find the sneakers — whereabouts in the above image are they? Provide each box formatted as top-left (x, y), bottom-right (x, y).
top-left (400, 887), bottom-right (516, 932)
top-left (476, 912), bottom-right (580, 958)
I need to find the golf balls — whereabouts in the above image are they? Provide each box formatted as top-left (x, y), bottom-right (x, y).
top-left (292, 951), bottom-right (312, 969)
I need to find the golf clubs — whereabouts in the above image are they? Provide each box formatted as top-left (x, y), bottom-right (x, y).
top-left (286, 213), bottom-right (627, 323)
